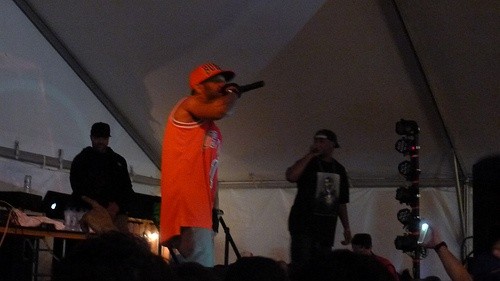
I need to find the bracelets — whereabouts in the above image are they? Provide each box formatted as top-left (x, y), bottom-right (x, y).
top-left (225, 89), bottom-right (240, 97)
top-left (434, 242), bottom-right (447, 252)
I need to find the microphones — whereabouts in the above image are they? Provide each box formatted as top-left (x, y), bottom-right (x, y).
top-left (223, 80), bottom-right (265, 97)
top-left (310, 147), bottom-right (320, 154)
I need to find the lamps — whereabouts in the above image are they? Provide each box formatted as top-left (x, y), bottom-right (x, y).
top-left (394, 118), bottom-right (421, 253)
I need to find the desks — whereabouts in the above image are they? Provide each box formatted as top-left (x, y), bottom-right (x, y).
top-left (0, 226), bottom-right (88, 281)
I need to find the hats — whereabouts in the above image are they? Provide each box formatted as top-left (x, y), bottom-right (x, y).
top-left (352, 233), bottom-right (372, 246)
top-left (313, 129), bottom-right (340, 148)
top-left (189, 63), bottom-right (235, 91)
top-left (90, 122), bottom-right (112, 138)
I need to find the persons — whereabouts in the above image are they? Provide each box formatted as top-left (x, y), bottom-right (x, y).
top-left (285, 128), bottom-right (352, 264)
top-left (352, 233), bottom-right (399, 281)
top-left (70, 122), bottom-right (134, 234)
top-left (412, 226), bottom-right (500, 281)
top-left (161, 62), bottom-right (240, 268)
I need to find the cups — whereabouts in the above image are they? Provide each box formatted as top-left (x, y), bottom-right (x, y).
top-left (24, 175), bottom-right (32, 194)
top-left (64, 210), bottom-right (78, 231)
